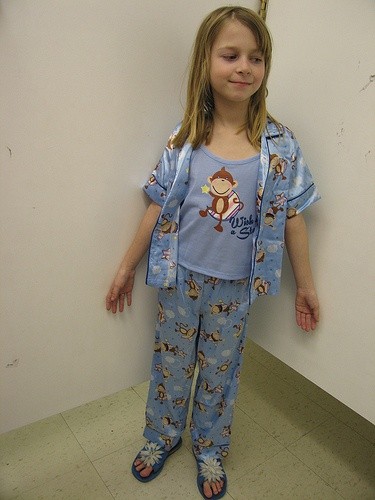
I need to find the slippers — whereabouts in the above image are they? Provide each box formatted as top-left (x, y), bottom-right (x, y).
top-left (133, 437), bottom-right (183, 481)
top-left (192, 445), bottom-right (227, 500)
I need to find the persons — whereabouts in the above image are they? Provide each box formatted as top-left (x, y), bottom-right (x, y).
top-left (105, 6), bottom-right (320, 500)
